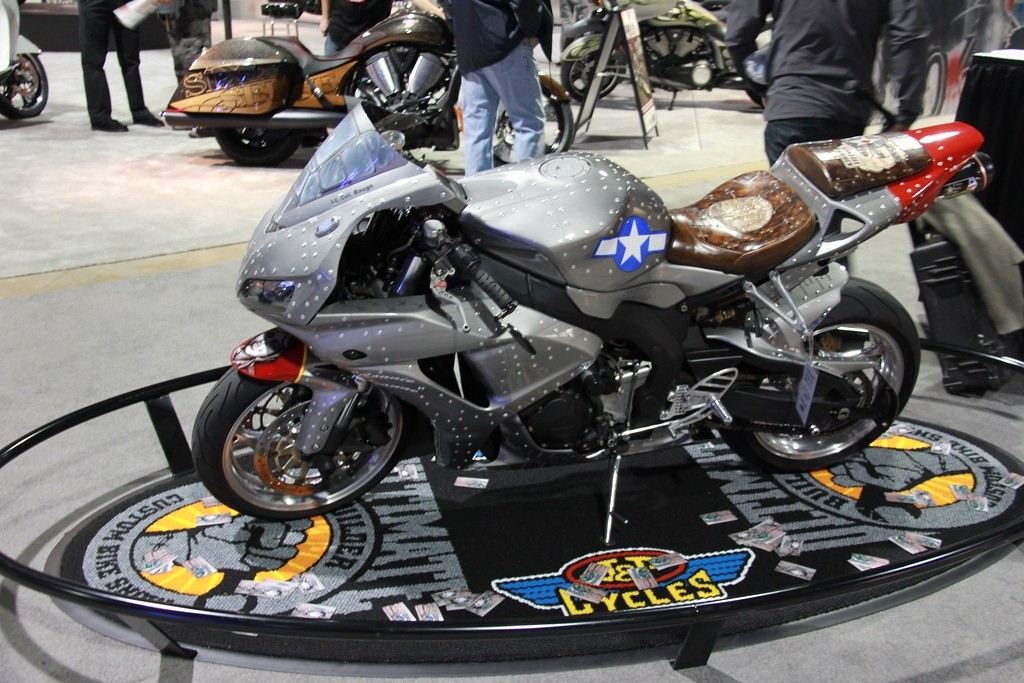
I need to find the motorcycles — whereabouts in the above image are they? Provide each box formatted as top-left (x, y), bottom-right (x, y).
top-left (0, 0), bottom-right (50, 120)
top-left (161, 1), bottom-right (994, 549)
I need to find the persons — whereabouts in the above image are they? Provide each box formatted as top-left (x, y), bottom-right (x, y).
top-left (723, 0), bottom-right (932, 275)
top-left (321, 0), bottom-right (446, 56)
top-left (437, 0), bottom-right (554, 177)
top-left (76, 0), bottom-right (218, 137)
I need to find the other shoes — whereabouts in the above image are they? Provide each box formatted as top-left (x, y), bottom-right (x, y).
top-left (91, 118), bottom-right (128, 131)
top-left (134, 116), bottom-right (164, 127)
